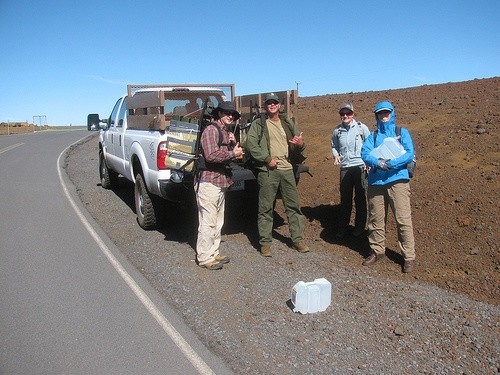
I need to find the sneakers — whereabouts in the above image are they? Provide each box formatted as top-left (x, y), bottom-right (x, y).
top-left (292, 240), bottom-right (309, 253)
top-left (215, 255), bottom-right (230, 264)
top-left (201, 260), bottom-right (223, 270)
top-left (260, 244), bottom-right (272, 256)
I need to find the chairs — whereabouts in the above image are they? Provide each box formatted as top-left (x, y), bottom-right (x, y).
top-left (174, 103), bottom-right (200, 116)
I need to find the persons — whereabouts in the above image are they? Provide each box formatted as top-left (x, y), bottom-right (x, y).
top-left (245, 92), bottom-right (310, 257)
top-left (360, 101), bottom-right (417, 274)
top-left (330, 103), bottom-right (371, 239)
top-left (193, 100), bottom-right (246, 270)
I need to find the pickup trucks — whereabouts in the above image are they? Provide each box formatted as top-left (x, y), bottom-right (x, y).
top-left (87, 84), bottom-right (311, 231)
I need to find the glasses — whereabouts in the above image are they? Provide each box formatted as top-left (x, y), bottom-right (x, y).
top-left (224, 111), bottom-right (235, 117)
top-left (340, 110), bottom-right (353, 116)
top-left (267, 100), bottom-right (279, 105)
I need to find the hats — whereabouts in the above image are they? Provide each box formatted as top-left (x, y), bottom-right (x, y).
top-left (217, 101), bottom-right (236, 113)
top-left (265, 93), bottom-right (280, 103)
top-left (376, 107), bottom-right (392, 113)
top-left (339, 102), bottom-right (354, 112)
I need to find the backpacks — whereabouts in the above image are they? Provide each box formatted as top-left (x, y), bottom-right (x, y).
top-left (374, 126), bottom-right (417, 178)
top-left (190, 114), bottom-right (232, 176)
top-left (244, 111), bottom-right (289, 168)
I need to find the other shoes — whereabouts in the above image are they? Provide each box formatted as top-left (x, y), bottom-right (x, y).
top-left (336, 227), bottom-right (347, 237)
top-left (352, 226), bottom-right (365, 236)
top-left (403, 260), bottom-right (415, 272)
top-left (362, 250), bottom-right (384, 266)
